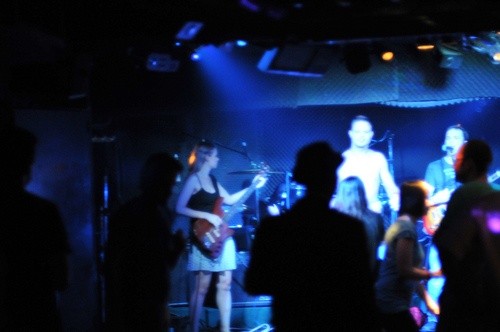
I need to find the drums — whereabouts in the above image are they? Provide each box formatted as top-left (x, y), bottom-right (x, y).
top-left (271, 181), bottom-right (307, 210)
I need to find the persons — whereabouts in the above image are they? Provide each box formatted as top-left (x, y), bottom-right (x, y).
top-left (0, 124), bottom-right (70, 332)
top-left (334, 115), bottom-right (400, 215)
top-left (241, 142), bottom-right (377, 332)
top-left (105, 153), bottom-right (184, 332)
top-left (423, 125), bottom-right (471, 332)
top-left (431, 141), bottom-right (500, 332)
top-left (378, 179), bottom-right (443, 332)
top-left (332, 176), bottom-right (384, 258)
top-left (177, 144), bottom-right (266, 332)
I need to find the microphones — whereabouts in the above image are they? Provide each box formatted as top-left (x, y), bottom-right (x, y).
top-left (442, 145), bottom-right (455, 152)
top-left (242, 142), bottom-right (257, 169)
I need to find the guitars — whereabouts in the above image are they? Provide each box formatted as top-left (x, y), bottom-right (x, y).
top-left (421, 169), bottom-right (500, 236)
top-left (189, 163), bottom-right (272, 261)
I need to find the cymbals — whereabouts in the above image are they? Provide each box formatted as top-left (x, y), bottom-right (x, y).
top-left (227, 169), bottom-right (287, 175)
